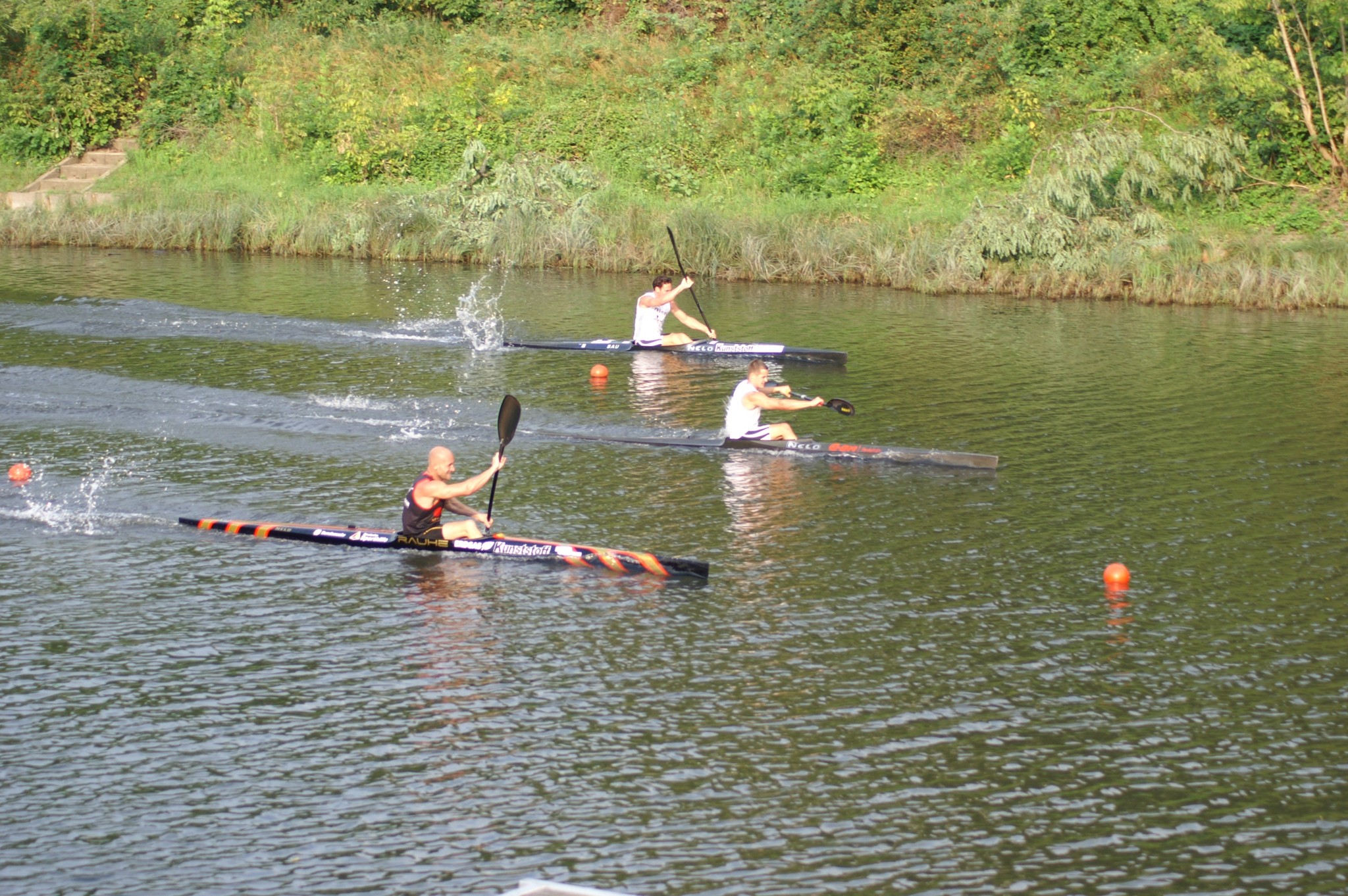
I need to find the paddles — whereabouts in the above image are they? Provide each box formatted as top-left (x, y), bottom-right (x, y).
top-left (765, 380), bottom-right (855, 416)
top-left (666, 226), bottom-right (716, 341)
top-left (484, 394), bottom-right (522, 536)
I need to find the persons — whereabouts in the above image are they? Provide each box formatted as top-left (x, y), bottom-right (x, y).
top-left (633, 275), bottom-right (716, 348)
top-left (724, 358), bottom-right (824, 441)
top-left (402, 446), bottom-right (507, 540)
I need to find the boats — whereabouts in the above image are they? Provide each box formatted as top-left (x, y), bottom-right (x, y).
top-left (179, 517), bottom-right (709, 581)
top-left (518, 427), bottom-right (999, 470)
top-left (503, 340), bottom-right (848, 364)
